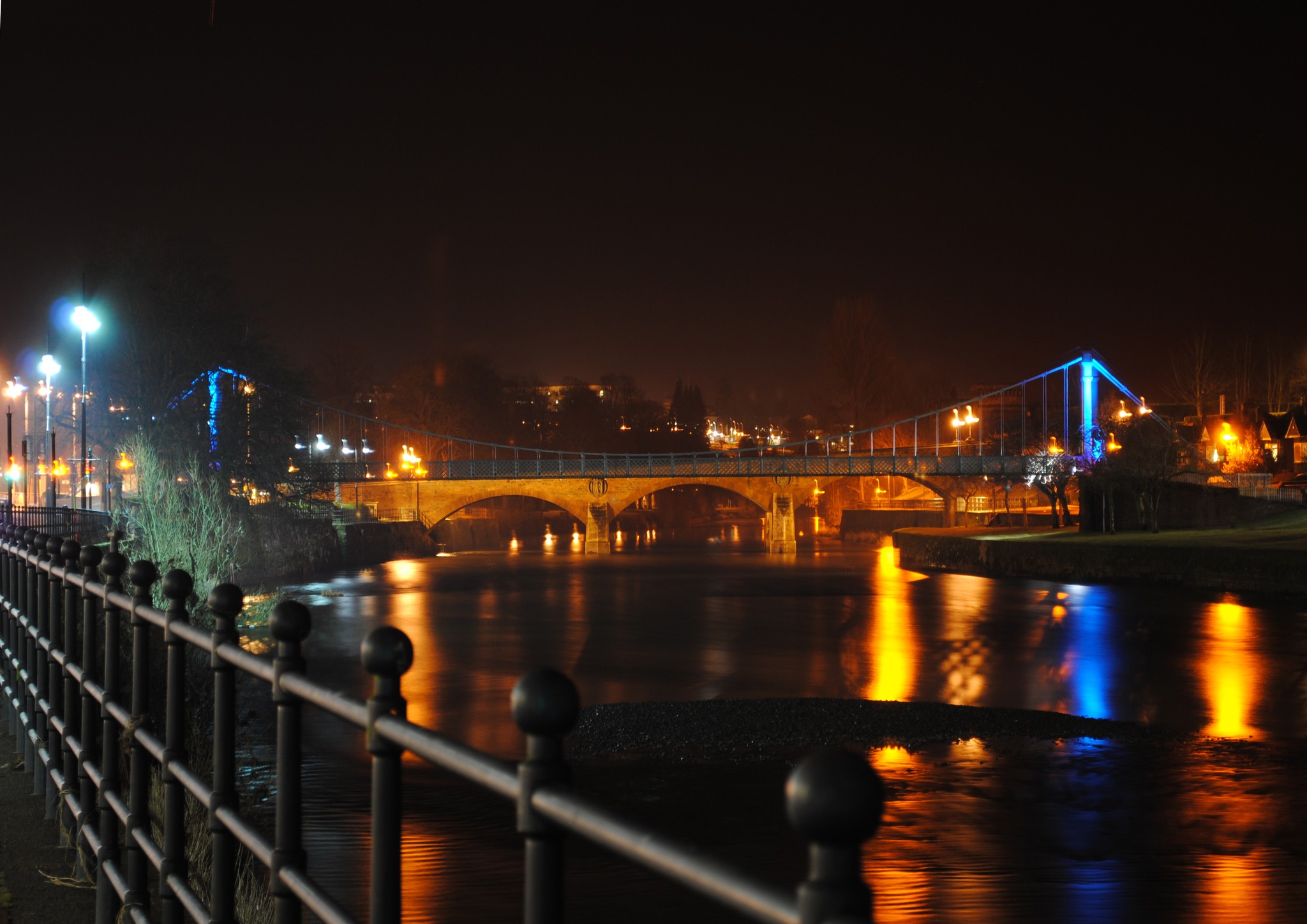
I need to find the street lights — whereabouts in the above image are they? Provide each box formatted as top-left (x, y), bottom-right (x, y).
top-left (2, 306), bottom-right (101, 526)
top-left (292, 433), bottom-right (373, 524)
